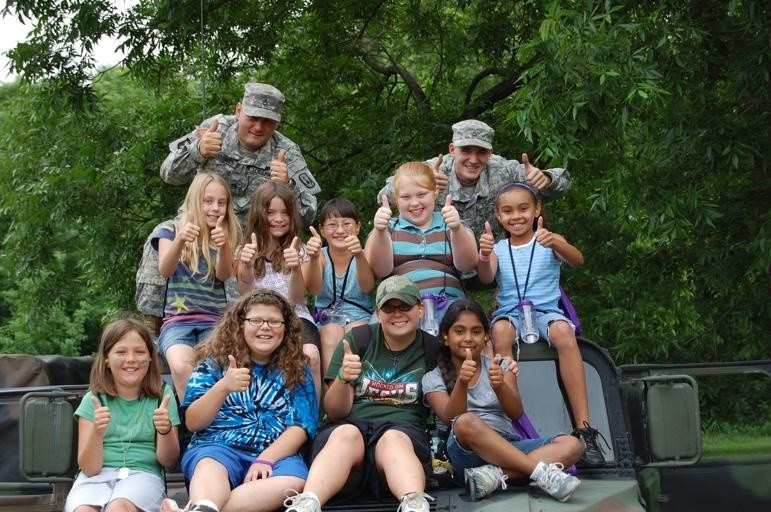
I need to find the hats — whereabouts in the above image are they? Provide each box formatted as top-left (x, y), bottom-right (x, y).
top-left (451, 120), bottom-right (495, 151)
top-left (375, 274), bottom-right (422, 312)
top-left (242, 82), bottom-right (286, 123)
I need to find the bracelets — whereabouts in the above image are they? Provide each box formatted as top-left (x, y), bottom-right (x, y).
top-left (251, 459), bottom-right (274, 468)
top-left (337, 367), bottom-right (351, 384)
top-left (479, 252), bottom-right (491, 262)
top-left (157, 419), bottom-right (172, 436)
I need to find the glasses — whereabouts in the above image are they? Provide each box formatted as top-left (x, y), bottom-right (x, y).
top-left (379, 302), bottom-right (419, 314)
top-left (239, 317), bottom-right (287, 329)
top-left (321, 222), bottom-right (359, 230)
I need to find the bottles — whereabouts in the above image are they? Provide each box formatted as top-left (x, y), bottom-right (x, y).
top-left (419, 293), bottom-right (440, 337)
top-left (519, 299), bottom-right (539, 343)
top-left (318, 307), bottom-right (355, 326)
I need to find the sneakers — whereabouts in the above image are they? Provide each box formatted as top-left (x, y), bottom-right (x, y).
top-left (569, 420), bottom-right (611, 468)
top-left (396, 491), bottom-right (435, 512)
top-left (184, 498), bottom-right (220, 512)
top-left (283, 488), bottom-right (322, 511)
top-left (537, 460), bottom-right (582, 503)
top-left (463, 465), bottom-right (510, 502)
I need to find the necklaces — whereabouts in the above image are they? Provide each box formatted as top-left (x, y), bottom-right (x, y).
top-left (118, 400), bottom-right (145, 479)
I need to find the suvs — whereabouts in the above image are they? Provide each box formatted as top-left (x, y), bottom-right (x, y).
top-left (17, 338), bottom-right (703, 512)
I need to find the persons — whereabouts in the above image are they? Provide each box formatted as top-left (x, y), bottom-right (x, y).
top-left (303, 197), bottom-right (377, 391)
top-left (64, 319), bottom-right (181, 512)
top-left (283, 274), bottom-right (444, 512)
top-left (376, 119), bottom-right (572, 279)
top-left (234, 181), bottom-right (323, 406)
top-left (150, 173), bottom-right (237, 405)
top-left (160, 288), bottom-right (319, 512)
top-left (135, 83), bottom-right (321, 339)
top-left (421, 297), bottom-right (585, 503)
top-left (476, 181), bottom-right (611, 469)
top-left (363, 161), bottom-right (479, 335)
